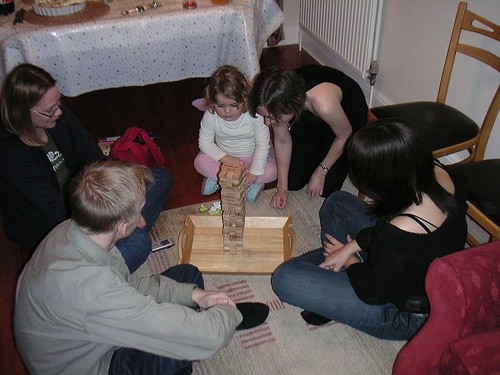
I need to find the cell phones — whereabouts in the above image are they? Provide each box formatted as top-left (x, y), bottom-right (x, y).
top-left (151, 237), bottom-right (175, 252)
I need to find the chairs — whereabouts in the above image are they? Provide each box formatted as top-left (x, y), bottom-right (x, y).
top-left (358, 1), bottom-right (500, 201)
top-left (439, 159), bottom-right (500, 247)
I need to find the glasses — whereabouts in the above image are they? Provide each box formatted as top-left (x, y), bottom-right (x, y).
top-left (30, 91), bottom-right (65, 119)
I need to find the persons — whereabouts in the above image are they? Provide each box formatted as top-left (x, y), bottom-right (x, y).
top-left (247, 65), bottom-right (369, 210)
top-left (0, 63), bottom-right (171, 275)
top-left (271, 118), bottom-right (469, 340)
top-left (13, 159), bottom-right (270, 375)
top-left (194, 64), bottom-right (277, 202)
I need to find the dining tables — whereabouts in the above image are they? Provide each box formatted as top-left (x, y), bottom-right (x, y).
top-left (0, 0), bottom-right (255, 99)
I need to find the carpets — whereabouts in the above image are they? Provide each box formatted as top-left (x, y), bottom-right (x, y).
top-left (129, 178), bottom-right (407, 375)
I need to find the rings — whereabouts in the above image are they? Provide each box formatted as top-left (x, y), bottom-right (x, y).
top-left (273, 202), bottom-right (276, 203)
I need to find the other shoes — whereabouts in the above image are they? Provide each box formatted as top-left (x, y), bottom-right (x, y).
top-left (200, 176), bottom-right (220, 196)
top-left (244, 183), bottom-right (264, 203)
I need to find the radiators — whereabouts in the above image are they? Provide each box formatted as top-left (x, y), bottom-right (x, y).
top-left (299, 0), bottom-right (384, 108)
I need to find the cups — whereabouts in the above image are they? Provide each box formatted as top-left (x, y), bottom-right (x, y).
top-left (182, 0), bottom-right (197, 9)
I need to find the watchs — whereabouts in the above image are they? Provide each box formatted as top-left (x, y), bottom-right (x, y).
top-left (320, 163), bottom-right (329, 171)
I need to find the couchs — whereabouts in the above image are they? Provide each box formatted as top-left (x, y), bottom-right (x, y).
top-left (392, 241), bottom-right (500, 375)
